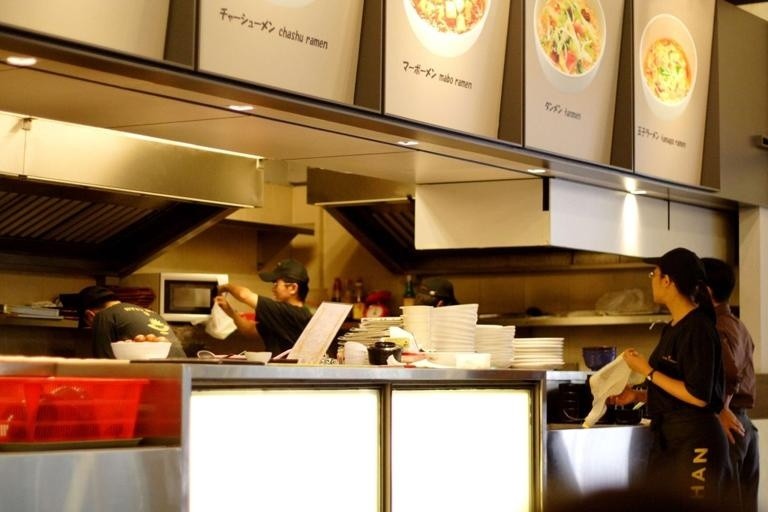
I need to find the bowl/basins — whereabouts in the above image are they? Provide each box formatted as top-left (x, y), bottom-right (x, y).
top-left (109, 341), bottom-right (172, 360)
top-left (533, 0), bottom-right (606, 94)
top-left (245, 351), bottom-right (273, 364)
top-left (406, 0), bottom-right (491, 58)
top-left (638, 11), bottom-right (700, 119)
top-left (583, 345), bottom-right (614, 370)
top-left (561, 382), bottom-right (643, 425)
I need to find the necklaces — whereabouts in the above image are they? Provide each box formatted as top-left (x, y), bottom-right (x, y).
top-left (213, 259), bottom-right (313, 358)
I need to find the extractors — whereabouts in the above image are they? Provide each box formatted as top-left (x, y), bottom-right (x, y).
top-left (306, 163), bottom-right (735, 277)
top-left (0, 110), bottom-right (263, 281)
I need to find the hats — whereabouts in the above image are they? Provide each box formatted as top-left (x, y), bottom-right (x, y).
top-left (418, 276), bottom-right (458, 303)
top-left (78, 286), bottom-right (112, 330)
top-left (259, 260), bottom-right (307, 283)
top-left (643, 249), bottom-right (706, 288)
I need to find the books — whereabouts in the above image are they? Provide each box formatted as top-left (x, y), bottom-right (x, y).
top-left (0, 303), bottom-right (59, 318)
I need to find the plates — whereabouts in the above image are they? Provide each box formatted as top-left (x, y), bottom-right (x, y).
top-left (400, 303), bottom-right (566, 372)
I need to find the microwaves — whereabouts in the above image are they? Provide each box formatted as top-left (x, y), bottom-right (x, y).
top-left (158, 270), bottom-right (229, 323)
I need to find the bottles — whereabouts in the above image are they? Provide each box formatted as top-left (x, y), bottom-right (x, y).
top-left (329, 277), bottom-right (343, 303)
top-left (401, 274), bottom-right (416, 308)
top-left (342, 275), bottom-right (367, 321)
top-left (370, 340), bottom-right (399, 364)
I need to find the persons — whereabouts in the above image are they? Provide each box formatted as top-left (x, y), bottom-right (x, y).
top-left (76, 285), bottom-right (188, 360)
top-left (605, 247), bottom-right (742, 511)
top-left (700, 258), bottom-right (759, 512)
top-left (413, 277), bottom-right (459, 308)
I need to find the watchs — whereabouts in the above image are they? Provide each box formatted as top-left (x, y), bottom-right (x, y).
top-left (646, 367), bottom-right (657, 382)
